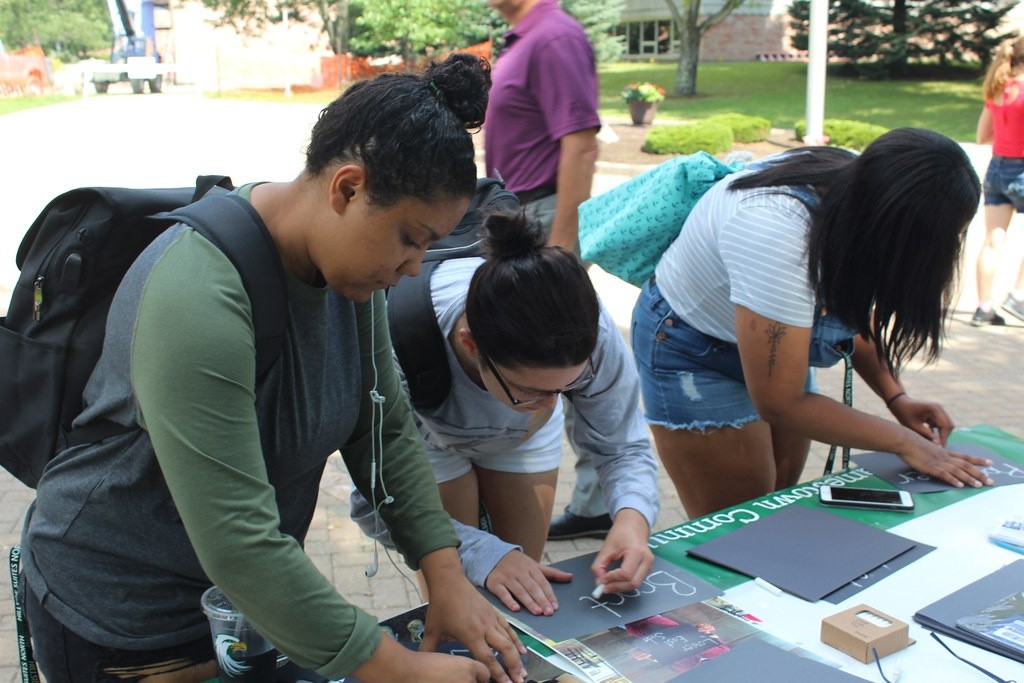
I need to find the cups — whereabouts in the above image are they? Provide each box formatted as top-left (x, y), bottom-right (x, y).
top-left (200, 585), bottom-right (296, 683)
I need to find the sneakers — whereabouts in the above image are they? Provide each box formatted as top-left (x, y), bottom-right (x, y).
top-left (971, 293), bottom-right (1024, 326)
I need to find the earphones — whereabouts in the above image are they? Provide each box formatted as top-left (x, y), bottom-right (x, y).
top-left (366, 551), bottom-right (379, 577)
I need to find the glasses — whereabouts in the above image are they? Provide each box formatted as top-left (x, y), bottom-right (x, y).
top-left (470, 332), bottom-right (596, 408)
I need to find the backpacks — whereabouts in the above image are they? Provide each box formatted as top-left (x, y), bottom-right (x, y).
top-left (1, 173), bottom-right (291, 491)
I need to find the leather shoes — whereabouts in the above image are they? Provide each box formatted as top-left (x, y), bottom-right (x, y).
top-left (546, 507), bottom-right (614, 539)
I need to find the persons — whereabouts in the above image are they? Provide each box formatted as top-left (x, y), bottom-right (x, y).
top-left (484, 0), bottom-right (615, 540)
top-left (20, 53), bottom-right (530, 683)
top-left (631, 127), bottom-right (996, 522)
top-left (970, 33), bottom-right (1024, 330)
top-left (349, 176), bottom-right (660, 616)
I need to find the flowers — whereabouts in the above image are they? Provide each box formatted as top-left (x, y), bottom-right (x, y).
top-left (620, 80), bottom-right (664, 103)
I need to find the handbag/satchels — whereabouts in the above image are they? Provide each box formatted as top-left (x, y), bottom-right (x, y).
top-left (577, 151), bottom-right (770, 288)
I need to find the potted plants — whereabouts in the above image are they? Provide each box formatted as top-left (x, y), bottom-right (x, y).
top-left (629, 104), bottom-right (655, 125)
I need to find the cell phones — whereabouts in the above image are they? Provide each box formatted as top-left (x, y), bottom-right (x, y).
top-left (819, 484), bottom-right (915, 513)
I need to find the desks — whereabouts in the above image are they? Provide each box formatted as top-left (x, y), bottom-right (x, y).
top-left (213, 432), bottom-right (1024, 683)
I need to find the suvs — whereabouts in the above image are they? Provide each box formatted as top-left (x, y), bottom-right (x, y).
top-left (92, 33), bottom-right (163, 94)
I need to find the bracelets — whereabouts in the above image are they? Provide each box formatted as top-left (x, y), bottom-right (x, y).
top-left (886, 391), bottom-right (906, 409)
